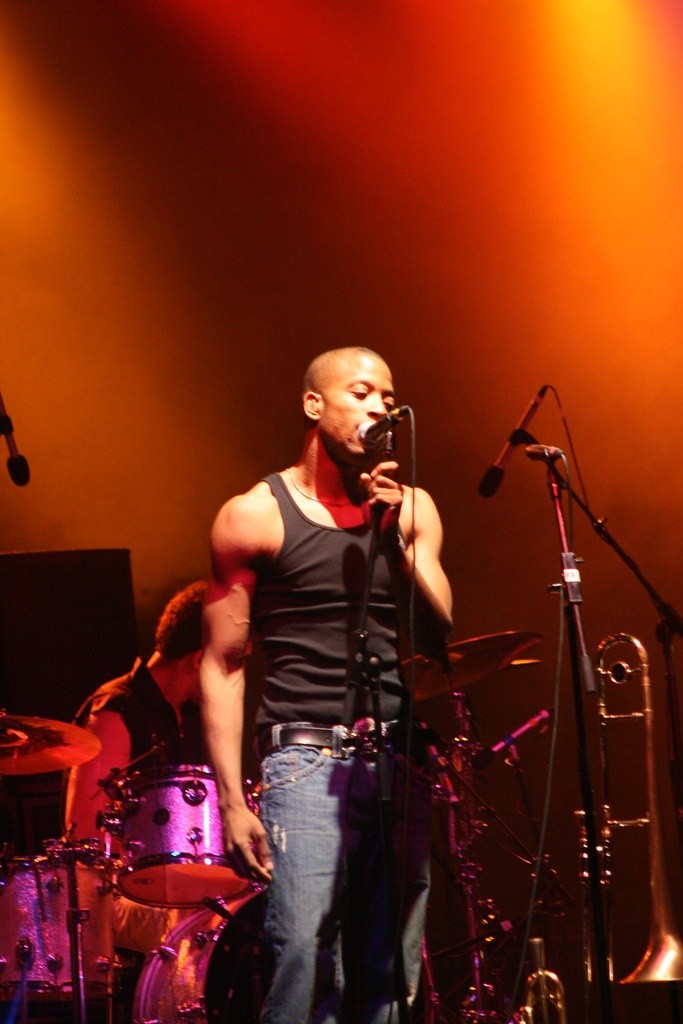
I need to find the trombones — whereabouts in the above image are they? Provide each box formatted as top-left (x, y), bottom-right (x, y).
top-left (573, 630), bottom-right (683, 1024)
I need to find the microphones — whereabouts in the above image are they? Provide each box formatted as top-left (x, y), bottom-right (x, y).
top-left (357, 406), bottom-right (411, 449)
top-left (479, 385), bottom-right (547, 499)
top-left (0, 395), bottom-right (30, 485)
top-left (491, 709), bottom-right (554, 753)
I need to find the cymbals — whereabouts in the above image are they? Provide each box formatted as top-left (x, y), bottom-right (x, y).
top-left (399, 628), bottom-right (548, 704)
top-left (0, 714), bottom-right (102, 775)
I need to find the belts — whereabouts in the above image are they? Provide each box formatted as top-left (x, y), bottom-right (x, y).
top-left (266, 728), bottom-right (379, 755)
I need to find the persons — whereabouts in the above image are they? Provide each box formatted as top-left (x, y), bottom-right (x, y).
top-left (197, 347), bottom-right (452, 1024)
top-left (58, 580), bottom-right (253, 856)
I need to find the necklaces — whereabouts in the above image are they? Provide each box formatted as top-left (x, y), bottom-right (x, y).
top-left (285, 469), bottom-right (353, 506)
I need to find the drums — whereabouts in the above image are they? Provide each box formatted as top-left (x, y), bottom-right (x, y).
top-left (132, 883), bottom-right (436, 1024)
top-left (0, 843), bottom-right (122, 1004)
top-left (105, 761), bottom-right (257, 911)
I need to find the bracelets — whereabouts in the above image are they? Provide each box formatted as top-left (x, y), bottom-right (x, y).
top-left (385, 533), bottom-right (405, 552)
